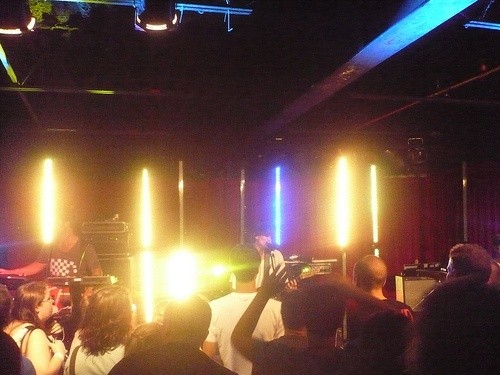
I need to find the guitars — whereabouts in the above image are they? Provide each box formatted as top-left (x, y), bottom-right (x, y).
top-left (256, 267), bottom-right (320, 299)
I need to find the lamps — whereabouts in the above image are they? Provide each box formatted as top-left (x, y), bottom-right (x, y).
top-left (0, 0), bottom-right (36, 39)
top-left (137, 0), bottom-right (181, 32)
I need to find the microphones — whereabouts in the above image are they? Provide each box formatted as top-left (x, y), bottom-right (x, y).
top-left (266, 240), bottom-right (282, 251)
top-left (76, 236), bottom-right (93, 278)
top-left (44, 239), bottom-right (54, 278)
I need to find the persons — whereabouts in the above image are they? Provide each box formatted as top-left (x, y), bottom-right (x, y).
top-left (0, 205), bottom-right (500, 375)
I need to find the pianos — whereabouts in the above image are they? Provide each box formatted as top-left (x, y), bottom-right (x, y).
top-left (0, 272), bottom-right (118, 288)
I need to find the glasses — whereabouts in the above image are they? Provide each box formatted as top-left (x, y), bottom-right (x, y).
top-left (42, 297), bottom-right (54, 303)
